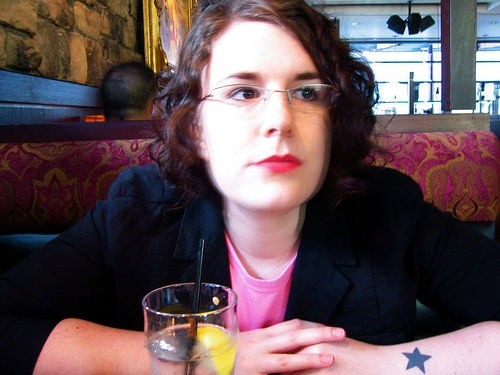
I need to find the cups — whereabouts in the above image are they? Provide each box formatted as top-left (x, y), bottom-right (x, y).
top-left (141, 282), bottom-right (238, 375)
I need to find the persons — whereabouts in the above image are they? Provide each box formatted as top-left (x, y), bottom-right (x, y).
top-left (97, 59), bottom-right (160, 124)
top-left (0, 0), bottom-right (500, 375)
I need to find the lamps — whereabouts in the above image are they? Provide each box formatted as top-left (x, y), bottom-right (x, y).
top-left (384, 0), bottom-right (435, 35)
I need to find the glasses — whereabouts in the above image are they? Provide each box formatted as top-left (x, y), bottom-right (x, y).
top-left (199, 83), bottom-right (337, 113)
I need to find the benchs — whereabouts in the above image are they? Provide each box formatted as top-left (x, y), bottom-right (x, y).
top-left (1, 112), bottom-right (500, 236)
